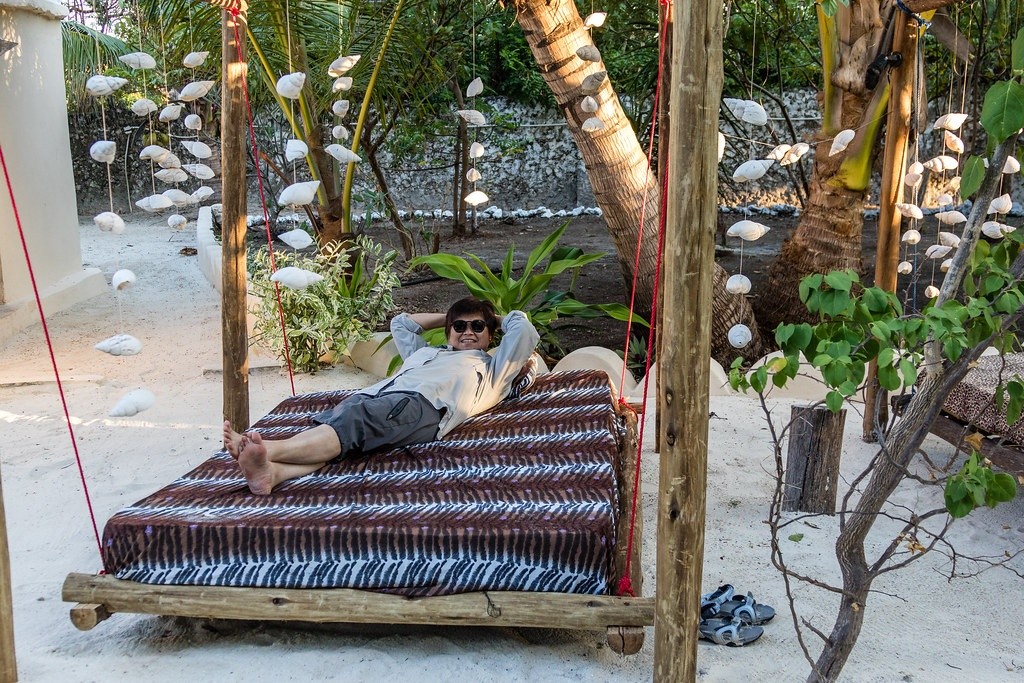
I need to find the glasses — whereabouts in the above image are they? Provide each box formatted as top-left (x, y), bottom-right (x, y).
top-left (450, 319), bottom-right (489, 333)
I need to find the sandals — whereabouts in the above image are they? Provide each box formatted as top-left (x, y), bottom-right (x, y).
top-left (701, 584), bottom-right (776, 626)
top-left (698, 601), bottom-right (763, 646)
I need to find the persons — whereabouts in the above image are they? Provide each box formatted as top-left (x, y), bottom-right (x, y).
top-left (224, 297), bottom-right (540, 495)
